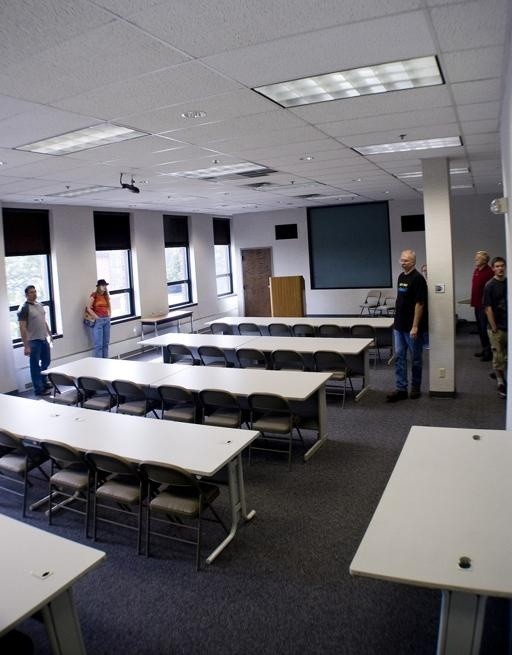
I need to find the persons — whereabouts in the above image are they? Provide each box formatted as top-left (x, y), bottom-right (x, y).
top-left (84, 278), bottom-right (111, 358)
top-left (387, 249), bottom-right (428, 402)
top-left (422, 264), bottom-right (427, 282)
top-left (16, 286), bottom-right (53, 395)
top-left (470, 252), bottom-right (495, 362)
top-left (481, 257), bottom-right (508, 398)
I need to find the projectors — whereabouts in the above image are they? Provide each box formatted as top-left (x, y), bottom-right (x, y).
top-left (122, 184), bottom-right (139, 193)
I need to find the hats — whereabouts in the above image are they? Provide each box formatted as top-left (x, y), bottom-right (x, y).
top-left (95, 279), bottom-right (110, 287)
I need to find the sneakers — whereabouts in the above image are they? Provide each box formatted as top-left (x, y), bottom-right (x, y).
top-left (473, 352), bottom-right (495, 362)
top-left (497, 381), bottom-right (507, 398)
top-left (385, 389), bottom-right (408, 401)
top-left (409, 385), bottom-right (421, 399)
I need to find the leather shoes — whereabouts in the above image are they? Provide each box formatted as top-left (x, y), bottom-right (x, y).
top-left (45, 385), bottom-right (53, 389)
top-left (34, 389), bottom-right (51, 395)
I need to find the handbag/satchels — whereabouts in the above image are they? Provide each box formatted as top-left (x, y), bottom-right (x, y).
top-left (83, 309), bottom-right (96, 329)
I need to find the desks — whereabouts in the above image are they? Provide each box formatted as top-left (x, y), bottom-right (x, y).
top-left (0, 515), bottom-right (106, 655)
top-left (348, 424), bottom-right (510, 655)
top-left (139, 310), bottom-right (194, 352)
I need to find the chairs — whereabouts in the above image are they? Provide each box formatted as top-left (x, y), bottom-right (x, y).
top-left (209, 323), bottom-right (381, 370)
top-left (41, 372), bottom-right (306, 471)
top-left (374, 291), bottom-right (397, 318)
top-left (358, 289), bottom-right (383, 317)
top-left (1, 430), bottom-right (231, 572)
top-left (165, 344), bottom-right (357, 411)
